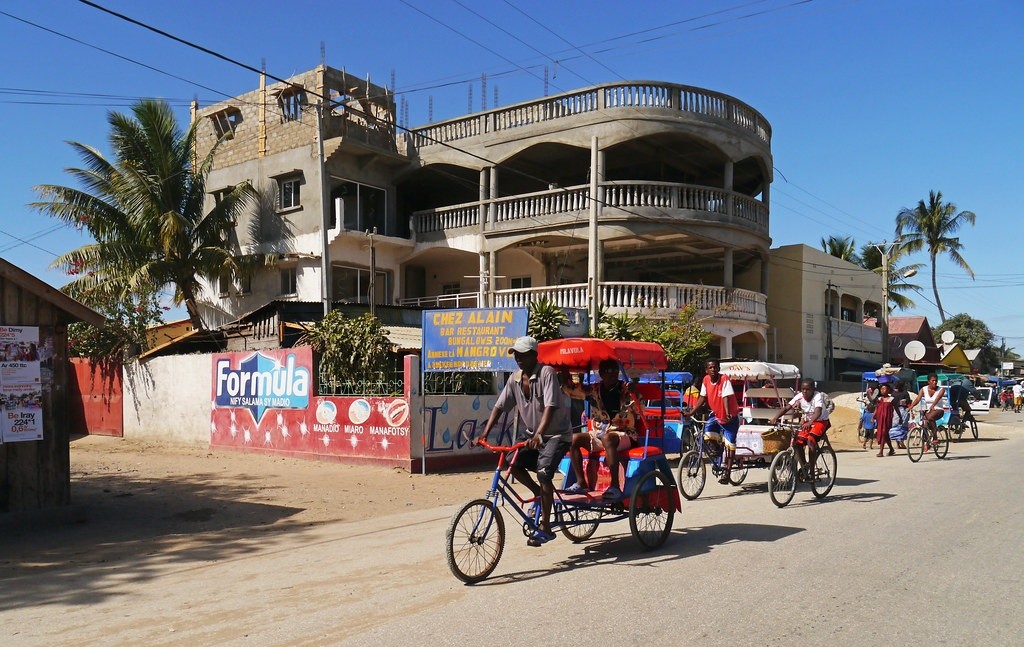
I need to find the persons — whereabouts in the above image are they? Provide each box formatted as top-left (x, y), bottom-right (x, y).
top-left (559, 356), bottom-right (639, 498)
top-left (0, 342), bottom-right (42, 410)
top-left (771, 377), bottom-right (832, 482)
top-left (758, 383), bottom-right (776, 409)
top-left (858, 382), bottom-right (915, 457)
top-left (473, 337), bottom-right (573, 545)
top-left (990, 380), bottom-right (1024, 414)
top-left (683, 377), bottom-right (701, 409)
top-left (948, 376), bottom-right (977, 434)
top-left (683, 356), bottom-right (739, 486)
top-left (906, 373), bottom-right (946, 454)
top-left (814, 381), bottom-right (835, 415)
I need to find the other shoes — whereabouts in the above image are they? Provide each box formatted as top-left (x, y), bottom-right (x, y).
top-left (901, 446), bottom-right (906, 449)
top-left (932, 440), bottom-right (939, 445)
top-left (897, 446), bottom-right (901, 449)
top-left (877, 453), bottom-right (883, 457)
top-left (863, 445), bottom-right (866, 449)
top-left (954, 430), bottom-right (960, 434)
top-left (799, 468), bottom-right (803, 473)
top-left (869, 446), bottom-right (873, 449)
top-left (808, 478), bottom-right (815, 484)
top-left (924, 445), bottom-right (930, 452)
top-left (712, 464), bottom-right (721, 471)
top-left (886, 451), bottom-right (895, 456)
top-left (961, 424), bottom-right (969, 428)
top-left (718, 476), bottom-right (730, 485)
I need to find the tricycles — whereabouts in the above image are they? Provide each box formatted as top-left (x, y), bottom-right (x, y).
top-left (672, 361), bottom-right (800, 501)
top-left (444, 339), bottom-right (681, 585)
top-left (856, 364), bottom-right (915, 443)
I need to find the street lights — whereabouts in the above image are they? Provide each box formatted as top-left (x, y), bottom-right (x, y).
top-left (365, 227), bottom-right (378, 324)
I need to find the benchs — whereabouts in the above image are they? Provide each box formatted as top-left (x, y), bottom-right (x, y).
top-left (639, 391), bottom-right (682, 423)
top-left (923, 396), bottom-right (959, 415)
top-left (566, 383), bottom-right (662, 460)
top-left (736, 388), bottom-right (796, 434)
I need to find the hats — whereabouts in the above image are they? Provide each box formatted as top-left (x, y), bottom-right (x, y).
top-left (508, 337), bottom-right (537, 355)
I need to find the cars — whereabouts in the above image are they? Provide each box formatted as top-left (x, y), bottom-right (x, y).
top-left (916, 373), bottom-right (1017, 417)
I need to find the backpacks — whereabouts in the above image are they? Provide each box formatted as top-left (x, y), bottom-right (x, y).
top-left (821, 392), bottom-right (835, 415)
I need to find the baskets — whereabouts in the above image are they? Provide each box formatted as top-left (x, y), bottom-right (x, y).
top-left (761, 429), bottom-right (792, 453)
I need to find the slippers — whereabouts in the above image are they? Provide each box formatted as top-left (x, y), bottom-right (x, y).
top-left (565, 483), bottom-right (589, 494)
top-left (602, 487), bottom-right (623, 498)
top-left (527, 502), bottom-right (538, 517)
top-left (533, 530), bottom-right (556, 543)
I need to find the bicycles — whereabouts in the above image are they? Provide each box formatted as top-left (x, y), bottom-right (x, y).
top-left (764, 420), bottom-right (838, 508)
top-left (907, 409), bottom-right (949, 464)
top-left (946, 397), bottom-right (979, 443)
